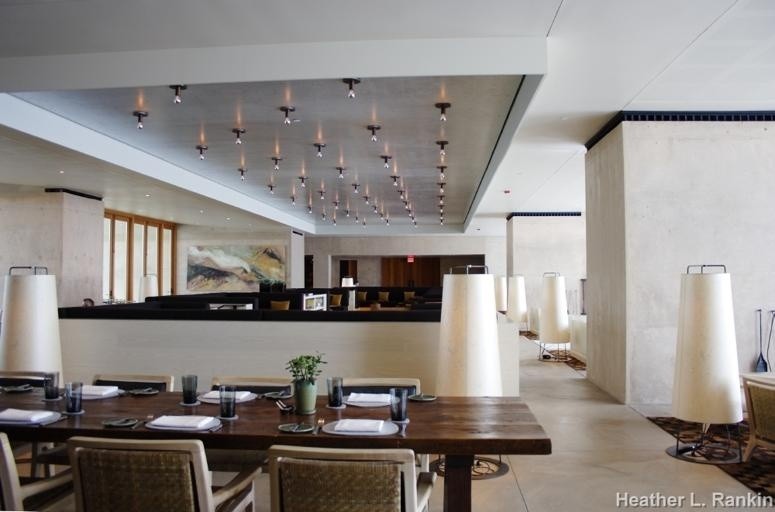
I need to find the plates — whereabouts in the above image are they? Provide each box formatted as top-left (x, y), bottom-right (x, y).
top-left (264, 391), bottom-right (294, 400)
top-left (323, 419), bottom-right (400, 437)
top-left (0, 408), bottom-right (63, 426)
top-left (147, 417), bottom-right (221, 431)
top-left (277, 424), bottom-right (314, 432)
top-left (198, 393), bottom-right (256, 404)
top-left (129, 388), bottom-right (160, 395)
top-left (409, 395), bottom-right (437, 401)
top-left (63, 389), bottom-right (125, 400)
top-left (104, 418), bottom-right (138, 427)
top-left (341, 395), bottom-right (400, 407)
top-left (4, 386), bottom-right (33, 392)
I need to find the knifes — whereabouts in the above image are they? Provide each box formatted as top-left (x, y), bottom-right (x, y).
top-left (208, 421), bottom-right (227, 432)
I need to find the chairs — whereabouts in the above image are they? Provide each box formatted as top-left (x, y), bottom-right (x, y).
top-left (91, 374), bottom-right (175, 396)
top-left (0, 371), bottom-right (59, 388)
top-left (67, 436), bottom-right (261, 512)
top-left (211, 375), bottom-right (291, 402)
top-left (0, 430), bottom-right (73, 512)
top-left (328, 376), bottom-right (429, 472)
top-left (254, 442), bottom-right (433, 512)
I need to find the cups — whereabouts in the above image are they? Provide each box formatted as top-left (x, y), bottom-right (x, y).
top-left (65, 383), bottom-right (82, 412)
top-left (327, 377), bottom-right (344, 408)
top-left (182, 375), bottom-right (197, 403)
top-left (391, 388), bottom-right (408, 421)
top-left (44, 371), bottom-right (59, 399)
top-left (219, 386), bottom-right (235, 418)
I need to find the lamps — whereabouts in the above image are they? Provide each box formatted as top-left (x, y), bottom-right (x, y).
top-left (537, 271), bottom-right (573, 362)
top-left (667, 264), bottom-right (748, 465)
top-left (495, 276), bottom-right (507, 311)
top-left (0, 263), bottom-right (62, 388)
top-left (436, 262), bottom-right (508, 481)
top-left (509, 277), bottom-right (529, 336)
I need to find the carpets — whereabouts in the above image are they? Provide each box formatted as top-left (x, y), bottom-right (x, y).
top-left (549, 350), bottom-right (585, 369)
top-left (519, 330), bottom-right (537, 340)
top-left (646, 414), bottom-right (775, 503)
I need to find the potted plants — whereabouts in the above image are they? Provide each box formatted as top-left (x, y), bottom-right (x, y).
top-left (284, 352), bottom-right (325, 415)
